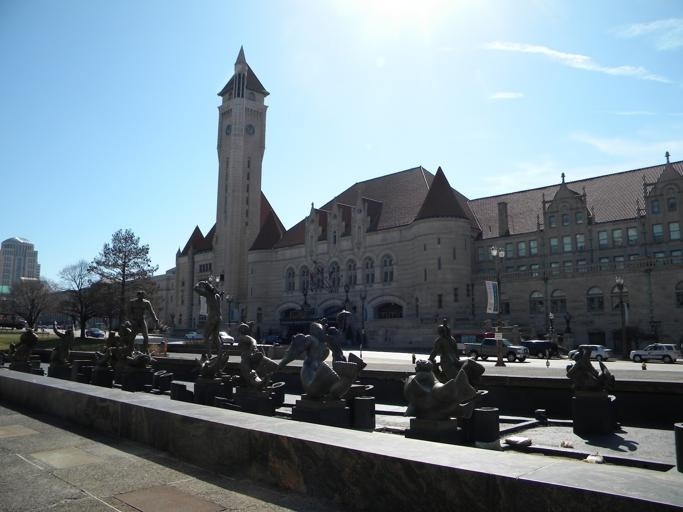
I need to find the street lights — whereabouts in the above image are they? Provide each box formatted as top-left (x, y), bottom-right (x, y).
top-left (490, 246), bottom-right (504, 368)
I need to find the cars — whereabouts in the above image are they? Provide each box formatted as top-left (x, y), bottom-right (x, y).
top-left (630, 343), bottom-right (680, 364)
top-left (568, 345), bottom-right (609, 362)
top-left (86, 328), bottom-right (104, 338)
top-left (185, 330), bottom-right (205, 341)
top-left (453, 331), bottom-right (495, 353)
top-left (218, 331), bottom-right (235, 346)
top-left (464, 337), bottom-right (529, 363)
top-left (521, 340), bottom-right (558, 359)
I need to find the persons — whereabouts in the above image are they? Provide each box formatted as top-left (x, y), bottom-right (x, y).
top-left (194, 280), bottom-right (225, 358)
top-left (127, 290), bottom-right (160, 361)
